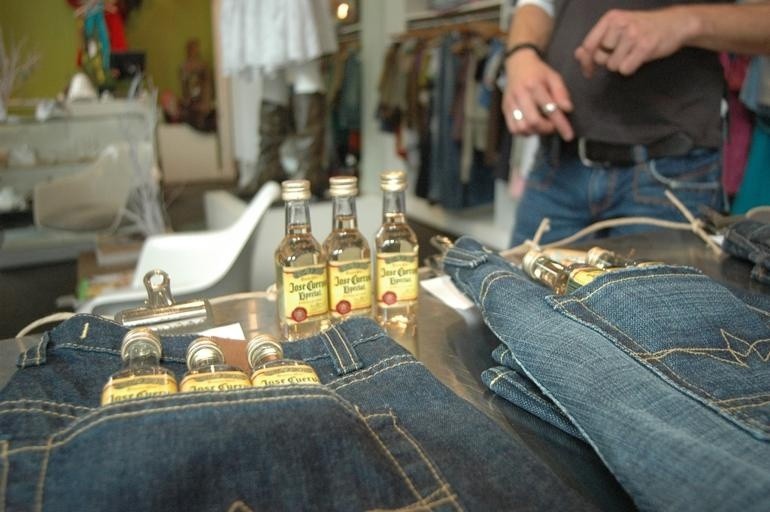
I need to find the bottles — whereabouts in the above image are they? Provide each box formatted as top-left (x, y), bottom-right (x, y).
top-left (243, 333), bottom-right (322, 390)
top-left (583, 245), bottom-right (667, 274)
top-left (179, 332), bottom-right (253, 397)
top-left (99, 324), bottom-right (178, 409)
top-left (322, 174), bottom-right (373, 329)
top-left (273, 178), bottom-right (330, 344)
top-left (519, 247), bottom-right (610, 301)
top-left (372, 168), bottom-right (419, 328)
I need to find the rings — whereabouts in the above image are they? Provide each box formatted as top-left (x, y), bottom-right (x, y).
top-left (601, 43), bottom-right (615, 57)
top-left (542, 102), bottom-right (558, 114)
top-left (512, 109), bottom-right (524, 121)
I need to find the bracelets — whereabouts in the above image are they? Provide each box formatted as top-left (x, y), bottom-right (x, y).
top-left (503, 43), bottom-right (543, 61)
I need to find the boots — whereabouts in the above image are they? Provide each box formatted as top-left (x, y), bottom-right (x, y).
top-left (237, 101), bottom-right (289, 201)
top-left (292, 93), bottom-right (329, 197)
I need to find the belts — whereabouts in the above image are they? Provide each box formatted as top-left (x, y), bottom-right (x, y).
top-left (560, 137), bottom-right (646, 169)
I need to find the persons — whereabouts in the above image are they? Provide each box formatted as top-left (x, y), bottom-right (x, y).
top-left (498, 1), bottom-right (769, 251)
top-left (222, 1), bottom-right (343, 202)
top-left (179, 39), bottom-right (214, 130)
